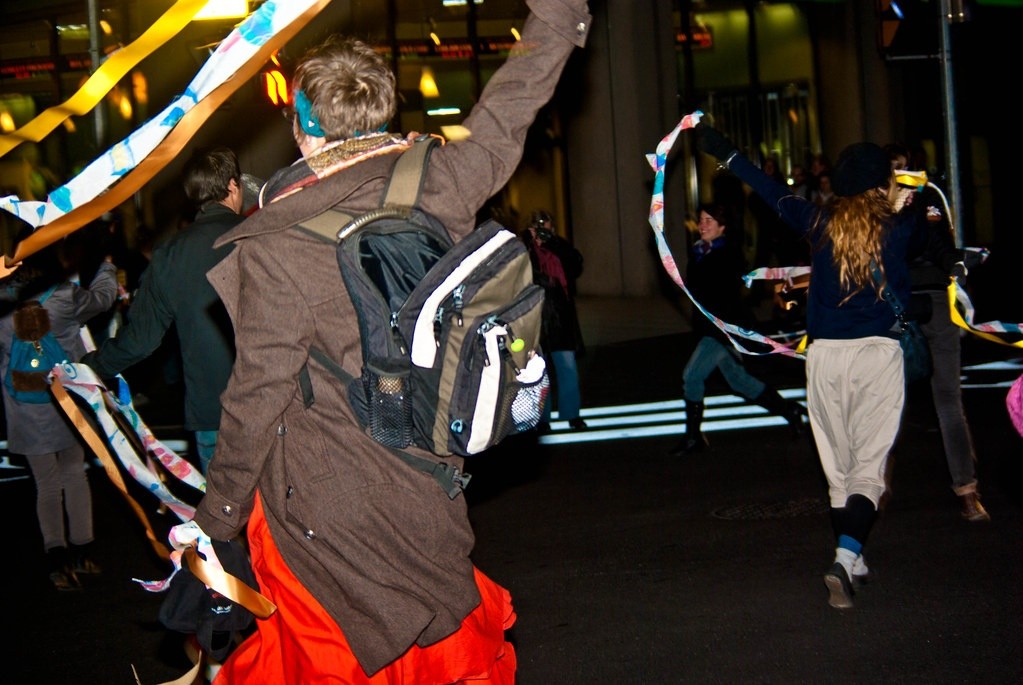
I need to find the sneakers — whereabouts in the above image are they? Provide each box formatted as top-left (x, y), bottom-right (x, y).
top-left (955, 478), bottom-right (990, 524)
top-left (822, 560), bottom-right (856, 612)
top-left (535, 422), bottom-right (550, 434)
top-left (48, 554), bottom-right (81, 593)
top-left (847, 554), bottom-right (871, 584)
top-left (62, 552), bottom-right (104, 575)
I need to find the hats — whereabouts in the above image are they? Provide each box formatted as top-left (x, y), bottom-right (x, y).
top-left (826, 142), bottom-right (895, 197)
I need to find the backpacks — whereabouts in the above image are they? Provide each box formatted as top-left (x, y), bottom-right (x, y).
top-left (7, 276), bottom-right (73, 407)
top-left (287, 132), bottom-right (554, 457)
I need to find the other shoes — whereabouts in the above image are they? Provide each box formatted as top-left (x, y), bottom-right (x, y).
top-left (568, 416), bottom-right (588, 430)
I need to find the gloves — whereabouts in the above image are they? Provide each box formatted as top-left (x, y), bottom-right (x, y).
top-left (688, 120), bottom-right (738, 165)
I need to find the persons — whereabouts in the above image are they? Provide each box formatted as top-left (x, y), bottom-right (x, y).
top-left (671, 194), bottom-right (810, 462)
top-left (680, 114), bottom-right (997, 613)
top-left (739, 148), bottom-right (836, 288)
top-left (1, 128), bottom-right (267, 644)
top-left (516, 208), bottom-right (588, 434)
top-left (882, 135), bottom-right (989, 523)
top-left (166, 0), bottom-right (593, 685)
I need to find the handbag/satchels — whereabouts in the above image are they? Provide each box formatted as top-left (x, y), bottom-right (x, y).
top-left (898, 319), bottom-right (937, 387)
top-left (156, 537), bottom-right (262, 665)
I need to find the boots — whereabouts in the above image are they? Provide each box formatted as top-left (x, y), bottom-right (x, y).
top-left (760, 388), bottom-right (811, 441)
top-left (665, 398), bottom-right (713, 459)
